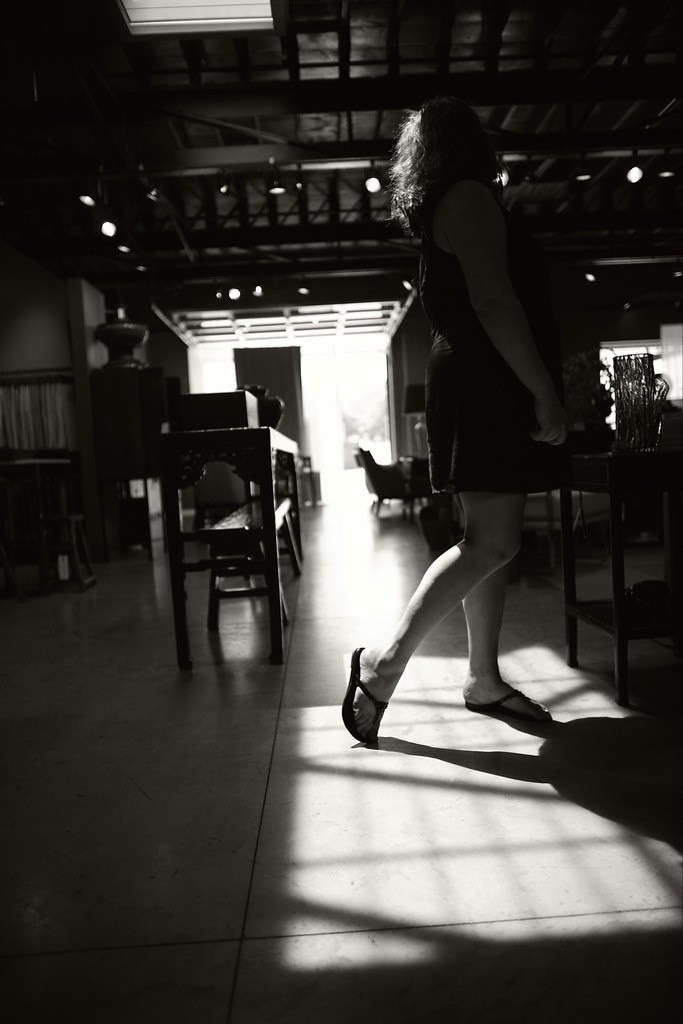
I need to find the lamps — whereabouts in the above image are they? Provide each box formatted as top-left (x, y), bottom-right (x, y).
top-left (625, 149), bottom-right (648, 184)
top-left (656, 147), bottom-right (678, 178)
top-left (78, 181), bottom-right (102, 206)
top-left (403, 383), bottom-right (427, 450)
top-left (267, 163), bottom-right (285, 194)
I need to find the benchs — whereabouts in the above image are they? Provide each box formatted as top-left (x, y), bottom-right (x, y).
top-left (207, 497), bottom-right (301, 631)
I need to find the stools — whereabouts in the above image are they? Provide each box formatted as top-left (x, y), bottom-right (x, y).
top-left (38, 513), bottom-right (95, 590)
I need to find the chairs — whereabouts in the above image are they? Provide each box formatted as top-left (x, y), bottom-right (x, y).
top-left (451, 488), bottom-right (611, 568)
top-left (358, 448), bottom-right (407, 520)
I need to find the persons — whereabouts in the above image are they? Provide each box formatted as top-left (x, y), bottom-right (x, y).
top-left (343, 97), bottom-right (572, 743)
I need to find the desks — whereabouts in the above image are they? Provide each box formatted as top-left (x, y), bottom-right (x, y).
top-left (0, 459), bottom-right (72, 596)
top-left (160, 427), bottom-right (301, 677)
top-left (560, 452), bottom-right (683, 706)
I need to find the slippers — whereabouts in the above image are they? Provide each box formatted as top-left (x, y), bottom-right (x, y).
top-left (464, 692), bottom-right (556, 724)
top-left (341, 646), bottom-right (388, 744)
